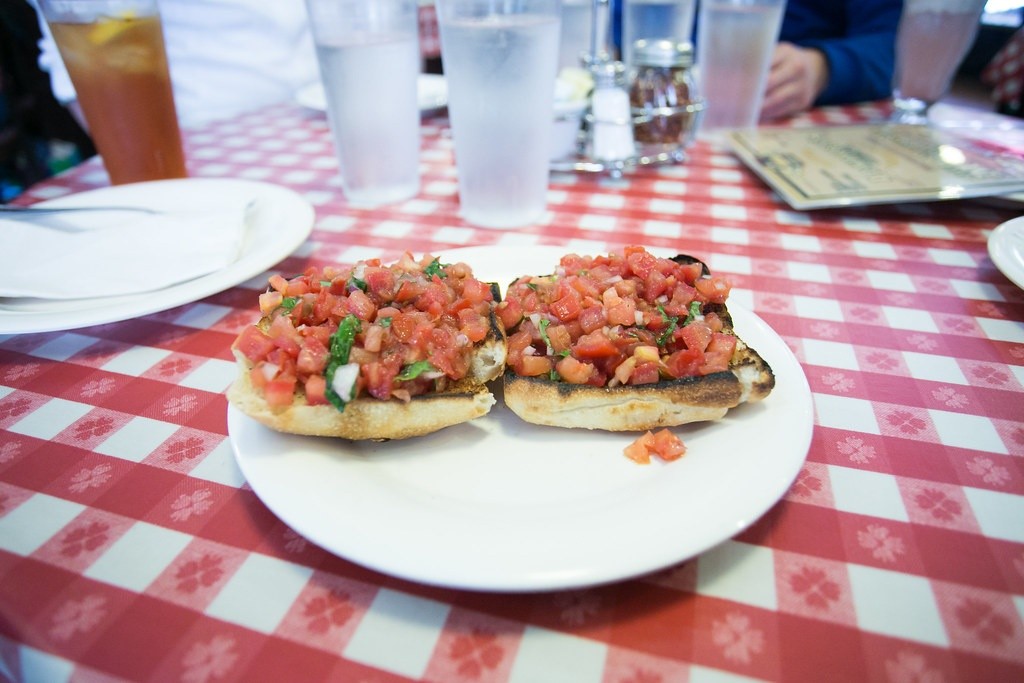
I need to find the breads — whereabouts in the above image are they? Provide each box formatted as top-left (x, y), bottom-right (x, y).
top-left (235, 275), bottom-right (508, 442)
top-left (503, 255), bottom-right (777, 432)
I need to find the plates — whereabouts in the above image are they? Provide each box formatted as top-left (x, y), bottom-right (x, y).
top-left (301, 72), bottom-right (447, 117)
top-left (0, 178), bottom-right (314, 335)
top-left (986, 215), bottom-right (1024, 290)
top-left (227, 246), bottom-right (815, 592)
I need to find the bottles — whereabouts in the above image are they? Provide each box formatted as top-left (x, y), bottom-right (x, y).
top-left (588, 62), bottom-right (635, 160)
top-left (629, 38), bottom-right (701, 164)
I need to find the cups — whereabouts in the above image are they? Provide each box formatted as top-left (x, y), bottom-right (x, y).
top-left (432, 0), bottom-right (562, 228)
top-left (301, 0), bottom-right (421, 209)
top-left (696, 0), bottom-right (788, 146)
top-left (883, 0), bottom-right (988, 126)
top-left (622, 0), bottom-right (695, 70)
top-left (549, 0), bottom-right (612, 179)
top-left (38, 0), bottom-right (188, 186)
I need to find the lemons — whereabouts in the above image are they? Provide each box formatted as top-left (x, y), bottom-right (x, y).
top-left (87, 20), bottom-right (151, 47)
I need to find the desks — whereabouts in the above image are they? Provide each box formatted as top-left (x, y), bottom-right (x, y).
top-left (0, 102), bottom-right (1024, 683)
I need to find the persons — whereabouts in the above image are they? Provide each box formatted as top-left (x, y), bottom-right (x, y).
top-left (27, 0), bottom-right (323, 130)
top-left (0, 66), bottom-right (85, 204)
top-left (610, 0), bottom-right (904, 120)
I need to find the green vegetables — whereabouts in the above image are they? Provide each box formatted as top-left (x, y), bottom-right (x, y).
top-left (278, 259), bottom-right (450, 414)
top-left (527, 272), bottom-right (701, 380)
